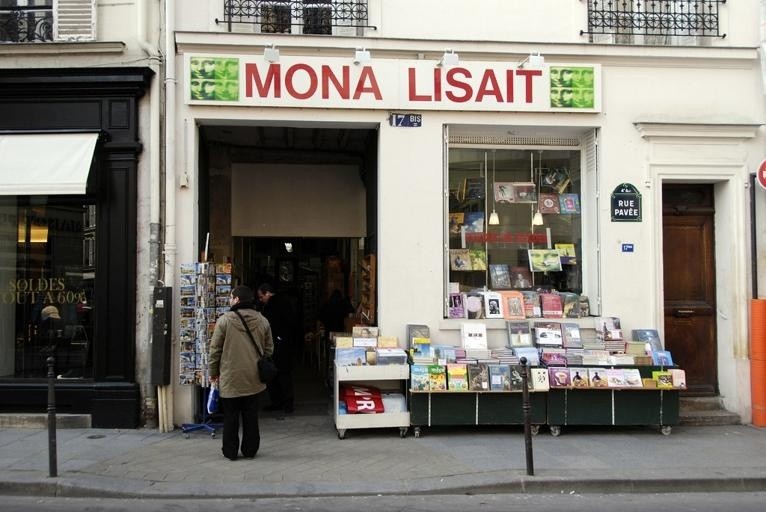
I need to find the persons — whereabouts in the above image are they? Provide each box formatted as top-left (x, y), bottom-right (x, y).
top-left (257, 283), bottom-right (297, 414)
top-left (207, 286), bottom-right (274, 460)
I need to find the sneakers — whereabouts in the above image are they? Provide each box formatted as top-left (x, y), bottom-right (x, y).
top-left (222, 446), bottom-right (255, 461)
top-left (262, 402), bottom-right (294, 413)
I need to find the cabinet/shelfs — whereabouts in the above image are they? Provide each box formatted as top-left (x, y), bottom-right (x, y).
top-left (334, 361), bottom-right (411, 438)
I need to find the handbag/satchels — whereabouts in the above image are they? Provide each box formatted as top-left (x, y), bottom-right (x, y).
top-left (206, 387), bottom-right (219, 415)
top-left (257, 356), bottom-right (279, 384)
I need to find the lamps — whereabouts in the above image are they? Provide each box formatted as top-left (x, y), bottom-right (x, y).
top-left (263, 43), bottom-right (544, 68)
top-left (489, 148), bottom-right (544, 226)
